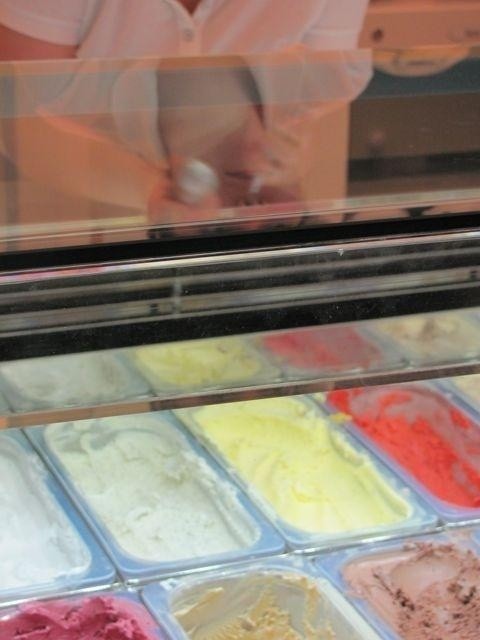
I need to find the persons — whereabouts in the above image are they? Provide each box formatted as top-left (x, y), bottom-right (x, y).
top-left (1, 0), bottom-right (378, 240)
